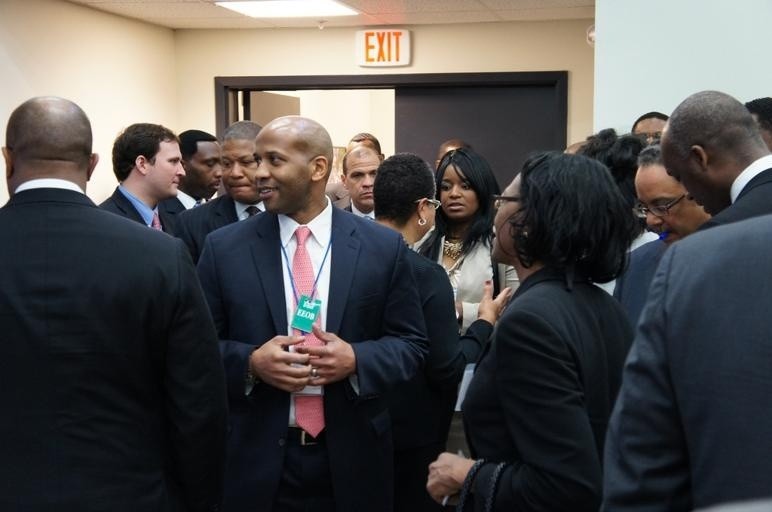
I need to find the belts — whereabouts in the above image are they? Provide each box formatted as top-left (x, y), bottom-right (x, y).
top-left (288, 428), bottom-right (326, 444)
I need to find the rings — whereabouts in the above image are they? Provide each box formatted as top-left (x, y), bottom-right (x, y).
top-left (312, 368), bottom-right (317, 376)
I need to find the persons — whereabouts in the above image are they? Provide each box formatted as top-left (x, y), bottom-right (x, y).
top-left (327, 90), bottom-right (772, 411)
top-left (197, 114), bottom-right (428, 512)
top-left (427, 150), bottom-right (637, 510)
top-left (0, 95), bottom-right (224, 511)
top-left (99, 121), bottom-right (266, 266)
top-left (604, 208), bottom-right (771, 511)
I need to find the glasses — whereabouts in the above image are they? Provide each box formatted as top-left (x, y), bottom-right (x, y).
top-left (492, 194), bottom-right (522, 210)
top-left (416, 198), bottom-right (442, 210)
top-left (632, 191), bottom-right (686, 219)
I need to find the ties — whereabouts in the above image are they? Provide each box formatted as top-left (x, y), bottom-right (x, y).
top-left (153, 214), bottom-right (164, 232)
top-left (292, 227), bottom-right (326, 439)
top-left (245, 206), bottom-right (261, 218)
